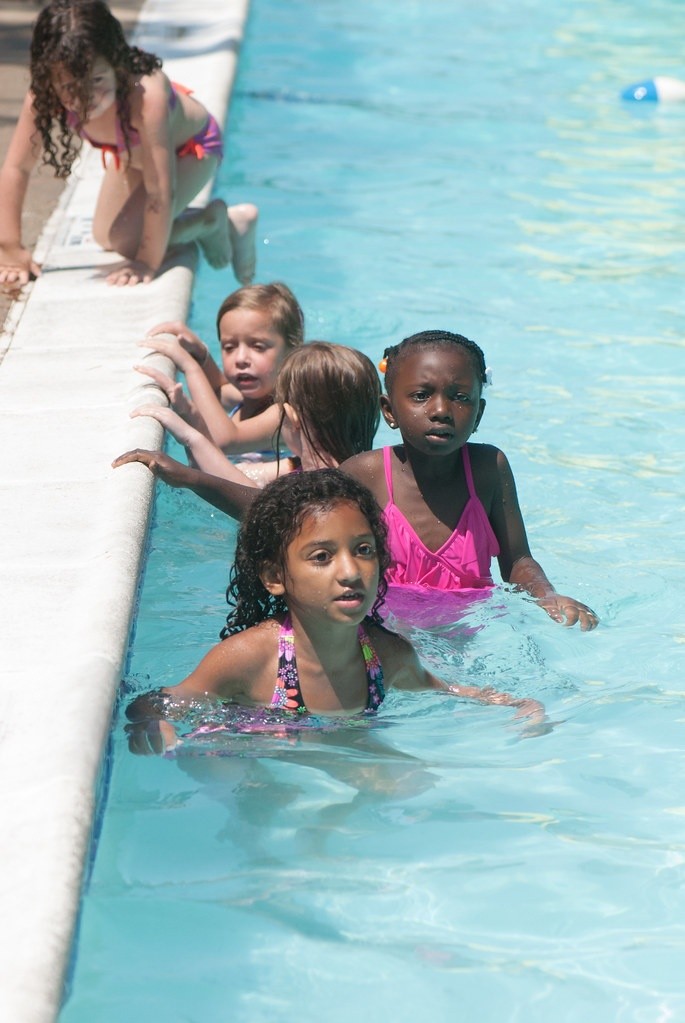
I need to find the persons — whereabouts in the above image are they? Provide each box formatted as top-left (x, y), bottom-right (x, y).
top-left (0, 0), bottom-right (597, 757)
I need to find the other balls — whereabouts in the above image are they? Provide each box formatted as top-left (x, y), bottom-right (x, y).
top-left (620, 76), bottom-right (685, 102)
